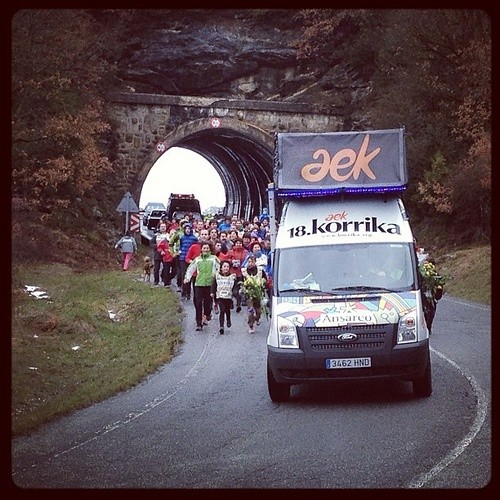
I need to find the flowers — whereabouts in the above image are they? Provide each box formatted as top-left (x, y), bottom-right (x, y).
top-left (419, 259), bottom-right (444, 309)
top-left (239, 273), bottom-right (272, 322)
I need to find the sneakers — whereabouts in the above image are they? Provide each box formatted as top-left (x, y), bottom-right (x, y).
top-left (196, 320), bottom-right (203, 331)
top-left (202, 315), bottom-right (209, 325)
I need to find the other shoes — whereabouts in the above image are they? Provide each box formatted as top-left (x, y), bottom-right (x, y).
top-left (236, 305), bottom-right (241, 313)
top-left (214, 304), bottom-right (220, 315)
top-left (226, 319), bottom-right (232, 328)
top-left (255, 316), bottom-right (261, 326)
top-left (219, 327), bottom-right (225, 334)
top-left (248, 325), bottom-right (255, 333)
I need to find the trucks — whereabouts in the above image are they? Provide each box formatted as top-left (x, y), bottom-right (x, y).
top-left (264, 128), bottom-right (435, 406)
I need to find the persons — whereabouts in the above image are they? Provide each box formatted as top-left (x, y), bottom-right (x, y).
top-left (365, 248), bottom-right (407, 280)
top-left (418, 258), bottom-right (443, 335)
top-left (148, 207), bottom-right (271, 336)
top-left (114, 231), bottom-right (137, 270)
